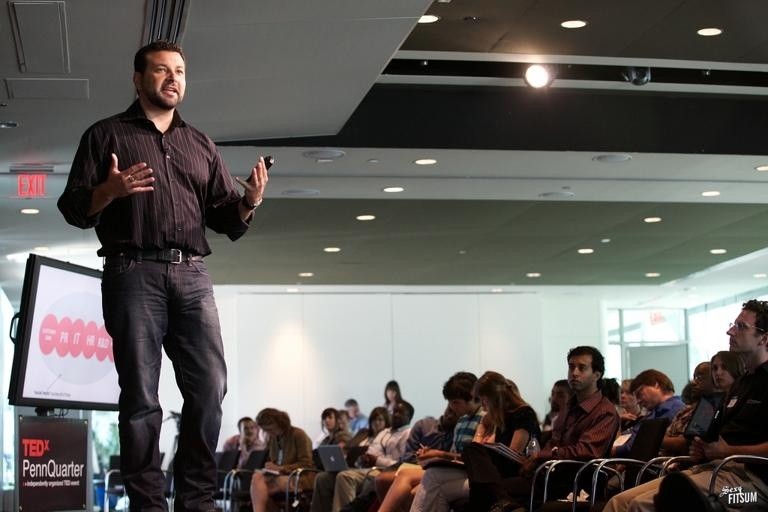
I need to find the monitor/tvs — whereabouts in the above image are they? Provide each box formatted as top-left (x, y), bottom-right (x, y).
top-left (8, 253), bottom-right (122, 411)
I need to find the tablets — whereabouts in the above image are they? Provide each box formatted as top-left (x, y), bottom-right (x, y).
top-left (683, 391), bottom-right (729, 442)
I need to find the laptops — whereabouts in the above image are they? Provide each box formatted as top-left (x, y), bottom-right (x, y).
top-left (317, 445), bottom-right (350, 472)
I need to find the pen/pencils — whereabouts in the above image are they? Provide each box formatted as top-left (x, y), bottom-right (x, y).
top-left (417, 440), bottom-right (427, 450)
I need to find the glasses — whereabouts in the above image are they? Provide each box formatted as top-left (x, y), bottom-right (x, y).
top-left (729, 321), bottom-right (765, 332)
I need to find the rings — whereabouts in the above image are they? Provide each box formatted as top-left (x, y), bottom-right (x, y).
top-left (128, 176), bottom-right (135, 182)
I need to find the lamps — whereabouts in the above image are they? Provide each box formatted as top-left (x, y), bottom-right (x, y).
top-left (623, 64), bottom-right (651, 85)
top-left (520, 60), bottom-right (559, 90)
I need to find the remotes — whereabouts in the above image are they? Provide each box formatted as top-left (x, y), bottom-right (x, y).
top-left (245, 156), bottom-right (275, 183)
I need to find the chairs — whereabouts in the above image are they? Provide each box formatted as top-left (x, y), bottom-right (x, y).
top-left (104, 445), bottom-right (768, 511)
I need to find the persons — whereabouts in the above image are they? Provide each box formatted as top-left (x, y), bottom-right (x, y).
top-left (57, 39), bottom-right (270, 512)
top-left (224, 299), bottom-right (767, 512)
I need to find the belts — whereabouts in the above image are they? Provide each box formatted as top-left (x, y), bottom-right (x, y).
top-left (96, 248), bottom-right (202, 266)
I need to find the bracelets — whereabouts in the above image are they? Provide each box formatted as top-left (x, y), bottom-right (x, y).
top-left (242, 196), bottom-right (262, 211)
